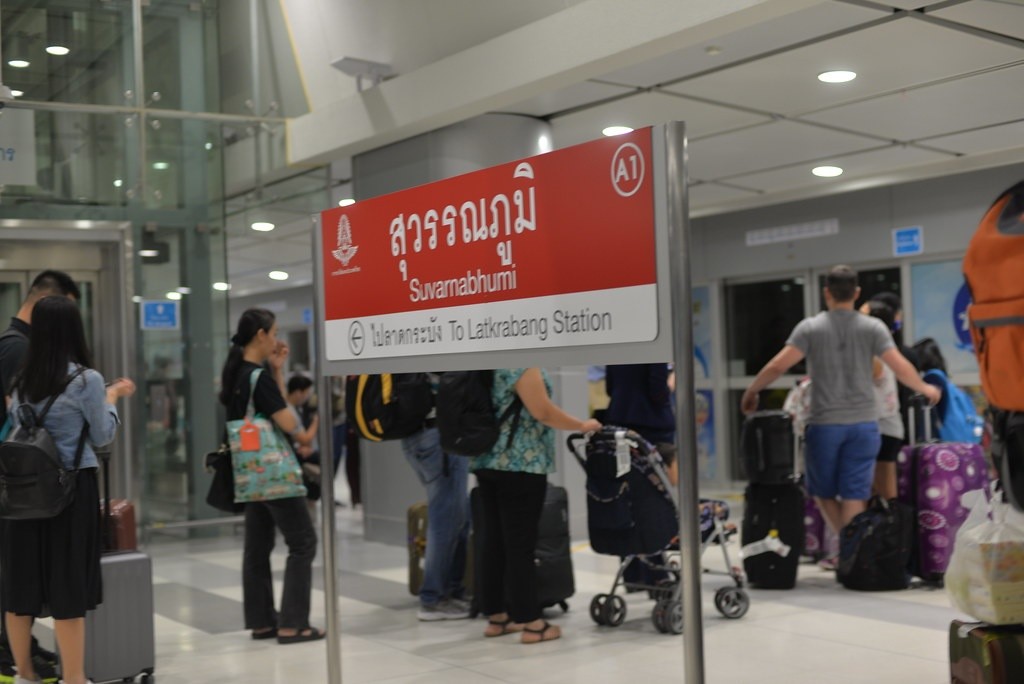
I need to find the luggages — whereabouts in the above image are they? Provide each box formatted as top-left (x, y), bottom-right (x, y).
top-left (407, 501), bottom-right (430, 595)
top-left (55, 553), bottom-right (155, 684)
top-left (898, 395), bottom-right (991, 587)
top-left (744, 410), bottom-right (804, 588)
top-left (787, 417), bottom-right (824, 565)
top-left (469, 484), bottom-right (573, 617)
top-left (93, 450), bottom-right (136, 552)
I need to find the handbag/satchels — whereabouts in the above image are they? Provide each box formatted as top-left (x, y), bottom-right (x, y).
top-left (948, 480), bottom-right (1024, 624)
top-left (205, 450), bottom-right (246, 513)
top-left (226, 368), bottom-right (308, 502)
top-left (837, 494), bottom-right (911, 593)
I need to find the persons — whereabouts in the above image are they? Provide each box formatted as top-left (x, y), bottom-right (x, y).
top-left (0, 270), bottom-right (135, 684)
top-left (743, 264), bottom-right (991, 593)
top-left (219, 307), bottom-right (675, 644)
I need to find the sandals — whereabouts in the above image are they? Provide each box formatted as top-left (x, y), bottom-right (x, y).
top-left (520, 622), bottom-right (560, 644)
top-left (483, 619), bottom-right (524, 636)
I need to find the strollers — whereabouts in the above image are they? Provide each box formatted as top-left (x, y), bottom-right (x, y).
top-left (566, 429), bottom-right (752, 634)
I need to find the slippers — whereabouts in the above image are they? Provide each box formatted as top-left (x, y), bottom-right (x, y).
top-left (253, 624), bottom-right (275, 638)
top-left (278, 627), bottom-right (325, 644)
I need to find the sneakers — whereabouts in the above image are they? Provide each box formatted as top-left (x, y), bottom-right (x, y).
top-left (418, 597), bottom-right (469, 620)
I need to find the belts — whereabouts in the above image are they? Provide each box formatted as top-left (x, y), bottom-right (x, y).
top-left (412, 418), bottom-right (436, 432)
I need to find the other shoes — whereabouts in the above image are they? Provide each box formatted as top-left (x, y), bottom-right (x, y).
top-left (0, 656), bottom-right (57, 684)
top-left (0, 636), bottom-right (57, 666)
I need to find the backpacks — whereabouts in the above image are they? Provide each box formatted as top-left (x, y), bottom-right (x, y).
top-left (434, 369), bottom-right (523, 456)
top-left (0, 366), bottom-right (89, 520)
top-left (351, 372), bottom-right (434, 442)
top-left (921, 371), bottom-right (985, 445)
